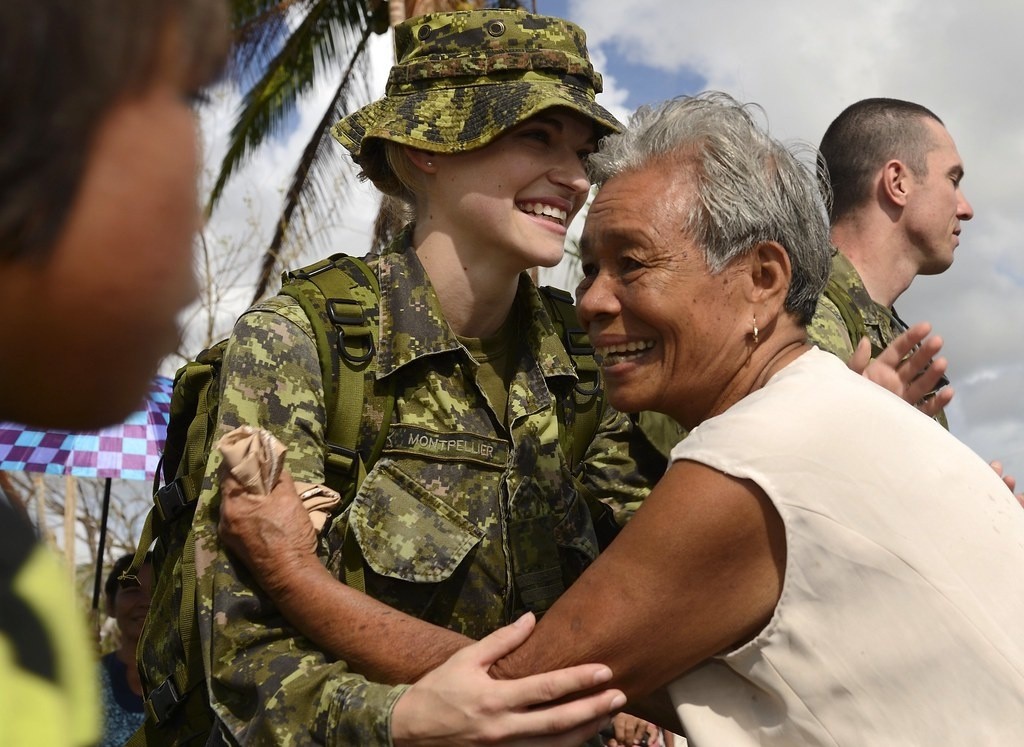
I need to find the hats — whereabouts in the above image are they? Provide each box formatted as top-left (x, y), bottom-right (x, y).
top-left (328, 7), bottom-right (627, 156)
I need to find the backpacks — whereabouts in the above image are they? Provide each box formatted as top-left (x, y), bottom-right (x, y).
top-left (123, 255), bottom-right (608, 745)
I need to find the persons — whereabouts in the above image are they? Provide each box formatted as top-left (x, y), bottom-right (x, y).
top-left (635, 98), bottom-right (975, 459)
top-left (191, 8), bottom-right (661, 747)
top-left (0, 0), bottom-right (237, 747)
top-left (217, 89), bottom-right (1024, 747)
top-left (96, 551), bottom-right (170, 747)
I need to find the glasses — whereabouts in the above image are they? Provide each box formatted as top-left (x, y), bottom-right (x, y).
top-left (116, 583), bottom-right (160, 598)
